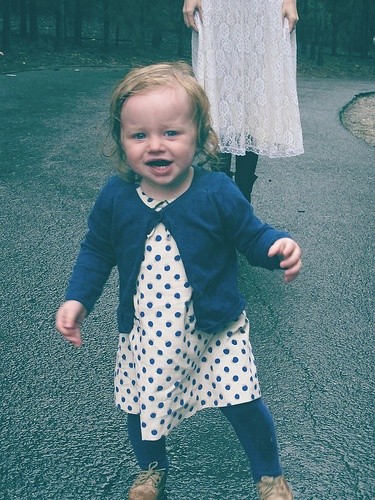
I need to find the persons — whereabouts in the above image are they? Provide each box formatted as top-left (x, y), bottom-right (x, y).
top-left (183, 0), bottom-right (299, 202)
top-left (55, 63), bottom-right (303, 500)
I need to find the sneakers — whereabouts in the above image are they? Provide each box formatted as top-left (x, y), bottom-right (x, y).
top-left (128, 462), bottom-right (167, 500)
top-left (256, 475), bottom-right (293, 500)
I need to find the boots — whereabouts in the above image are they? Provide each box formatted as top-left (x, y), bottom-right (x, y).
top-left (234, 172), bottom-right (258, 204)
top-left (212, 170), bottom-right (233, 179)
